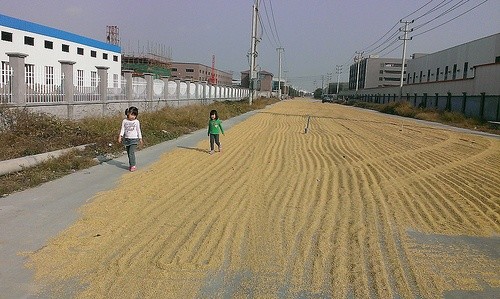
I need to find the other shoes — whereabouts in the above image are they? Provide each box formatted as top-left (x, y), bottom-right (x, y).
top-left (219, 144), bottom-right (222, 151)
top-left (209, 150), bottom-right (216, 154)
top-left (130, 166), bottom-right (136, 171)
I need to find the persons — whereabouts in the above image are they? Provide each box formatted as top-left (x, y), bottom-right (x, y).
top-left (207, 110), bottom-right (226, 155)
top-left (118, 106), bottom-right (144, 172)
